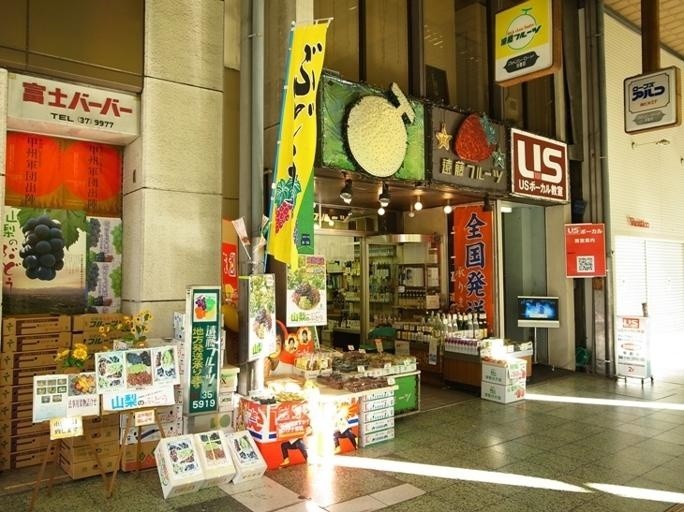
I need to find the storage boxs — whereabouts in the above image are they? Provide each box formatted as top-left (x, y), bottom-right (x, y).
top-left (193, 430), bottom-right (236, 490)
top-left (3, 311), bottom-right (240, 479)
top-left (358, 390), bottom-right (395, 450)
top-left (480, 359), bottom-right (527, 387)
top-left (224, 430), bottom-right (267, 485)
top-left (153, 434), bottom-right (204, 499)
top-left (479, 380), bottom-right (525, 403)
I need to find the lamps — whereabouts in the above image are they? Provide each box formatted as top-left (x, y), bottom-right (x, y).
top-left (337, 170), bottom-right (353, 205)
top-left (376, 179), bottom-right (392, 209)
top-left (408, 205), bottom-right (413, 217)
top-left (377, 206), bottom-right (386, 215)
top-left (443, 198), bottom-right (451, 215)
top-left (413, 195), bottom-right (422, 212)
top-left (481, 194), bottom-right (494, 213)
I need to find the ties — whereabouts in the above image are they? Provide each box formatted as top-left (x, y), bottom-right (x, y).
top-left (517, 295), bottom-right (560, 328)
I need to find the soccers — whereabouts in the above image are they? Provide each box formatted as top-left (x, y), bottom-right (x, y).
top-left (513, 387), bottom-right (526, 398)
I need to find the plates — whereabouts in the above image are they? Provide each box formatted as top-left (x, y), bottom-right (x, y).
top-left (392, 338), bottom-right (444, 387)
top-left (326, 256), bottom-right (397, 329)
top-left (442, 346), bottom-right (533, 393)
top-left (333, 321), bottom-right (374, 350)
top-left (335, 354), bottom-right (422, 422)
top-left (392, 263), bottom-right (441, 319)
top-left (238, 380), bottom-right (401, 470)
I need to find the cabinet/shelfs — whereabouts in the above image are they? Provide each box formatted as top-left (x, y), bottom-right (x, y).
top-left (392, 263), bottom-right (441, 319)
top-left (335, 354), bottom-right (422, 422)
top-left (326, 256), bottom-right (397, 329)
top-left (392, 338), bottom-right (444, 387)
top-left (442, 346), bottom-right (533, 393)
top-left (333, 321), bottom-right (374, 350)
top-left (238, 380), bottom-right (401, 470)
top-left (325, 254), bottom-right (492, 357)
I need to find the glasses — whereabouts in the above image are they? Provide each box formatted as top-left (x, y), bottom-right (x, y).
top-left (443, 198), bottom-right (451, 215)
top-left (337, 170), bottom-right (353, 205)
top-left (408, 205), bottom-right (413, 217)
top-left (481, 194), bottom-right (494, 213)
top-left (377, 206), bottom-right (386, 215)
top-left (413, 195), bottom-right (422, 212)
top-left (376, 179), bottom-right (392, 209)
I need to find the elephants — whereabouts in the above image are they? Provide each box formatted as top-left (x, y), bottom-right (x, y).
top-left (456, 114), bottom-right (498, 162)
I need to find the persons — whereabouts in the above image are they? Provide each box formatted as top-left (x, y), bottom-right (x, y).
top-left (264, 327), bottom-right (314, 378)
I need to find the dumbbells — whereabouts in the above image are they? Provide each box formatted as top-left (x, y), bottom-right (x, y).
top-left (219, 413), bottom-right (231, 428)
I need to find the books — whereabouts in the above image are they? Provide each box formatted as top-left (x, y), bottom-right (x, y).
top-left (358, 390), bottom-right (395, 450)
top-left (480, 359), bottom-right (527, 387)
top-left (479, 380), bottom-right (525, 403)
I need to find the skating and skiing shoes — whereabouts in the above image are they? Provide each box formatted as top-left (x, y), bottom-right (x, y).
top-left (195, 295), bottom-right (215, 319)
top-left (127, 350), bottom-right (152, 386)
top-left (109, 265), bottom-right (122, 297)
top-left (247, 277), bottom-right (275, 312)
top-left (18, 216), bottom-right (64, 280)
top-left (86, 262), bottom-right (99, 292)
top-left (111, 222), bottom-right (123, 254)
top-left (94, 295), bottom-right (103, 307)
top-left (95, 251), bottom-right (104, 262)
top-left (86, 218), bottom-right (101, 247)
top-left (275, 202), bottom-right (293, 233)
